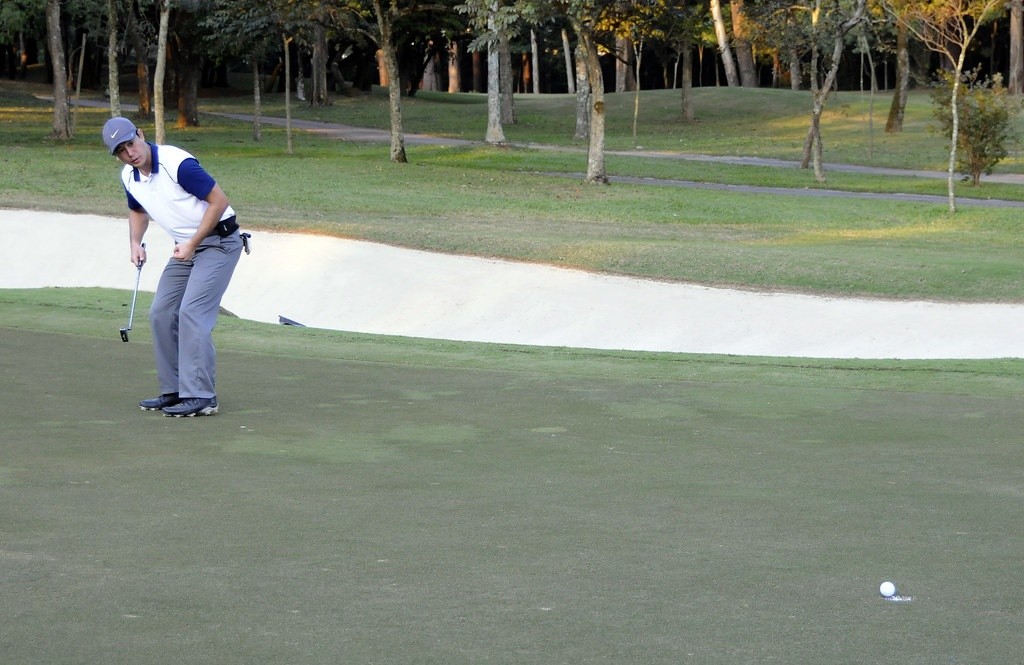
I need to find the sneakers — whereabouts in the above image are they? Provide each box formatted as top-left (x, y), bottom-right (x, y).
top-left (161, 396), bottom-right (219, 419)
top-left (138, 393), bottom-right (183, 411)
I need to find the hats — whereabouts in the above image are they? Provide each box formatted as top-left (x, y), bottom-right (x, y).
top-left (102, 117), bottom-right (137, 155)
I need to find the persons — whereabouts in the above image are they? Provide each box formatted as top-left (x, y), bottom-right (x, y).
top-left (102, 116), bottom-right (244, 417)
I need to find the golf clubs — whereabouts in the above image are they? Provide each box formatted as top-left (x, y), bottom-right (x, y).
top-left (119, 242), bottom-right (147, 342)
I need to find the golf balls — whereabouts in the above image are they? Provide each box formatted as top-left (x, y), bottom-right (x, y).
top-left (880, 581), bottom-right (897, 597)
